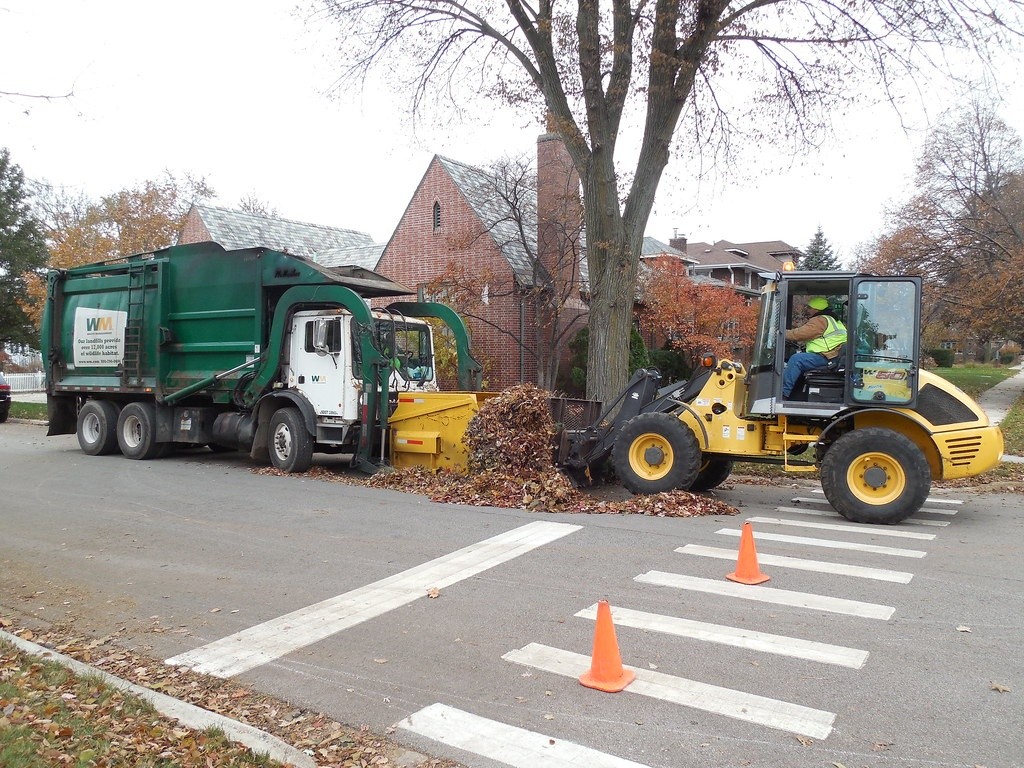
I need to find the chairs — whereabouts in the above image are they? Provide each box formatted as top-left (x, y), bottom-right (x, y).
top-left (803, 305), bottom-right (873, 388)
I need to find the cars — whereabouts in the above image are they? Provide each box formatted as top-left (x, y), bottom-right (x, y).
top-left (0, 371), bottom-right (11, 424)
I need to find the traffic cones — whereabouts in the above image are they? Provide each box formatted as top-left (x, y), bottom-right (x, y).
top-left (725, 521), bottom-right (771, 586)
top-left (578, 599), bottom-right (637, 693)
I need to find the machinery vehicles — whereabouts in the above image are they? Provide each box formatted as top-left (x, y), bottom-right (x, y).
top-left (40, 241), bottom-right (485, 474)
top-left (540, 269), bottom-right (1003, 526)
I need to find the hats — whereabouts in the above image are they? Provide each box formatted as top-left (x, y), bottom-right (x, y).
top-left (807, 297), bottom-right (829, 310)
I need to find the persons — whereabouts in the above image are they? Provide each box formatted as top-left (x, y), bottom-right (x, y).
top-left (779, 298), bottom-right (847, 400)
